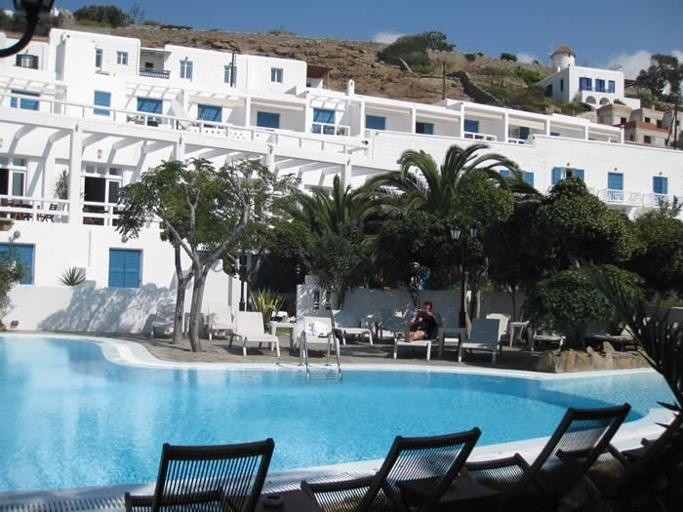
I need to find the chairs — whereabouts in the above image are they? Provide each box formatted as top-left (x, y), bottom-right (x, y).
top-left (1, 199), bottom-right (58, 222)
top-left (127, 437), bottom-right (276, 509)
top-left (555, 414), bottom-right (681, 510)
top-left (300, 427), bottom-right (483, 511)
top-left (151, 302), bottom-right (567, 363)
top-left (459, 403), bottom-right (634, 510)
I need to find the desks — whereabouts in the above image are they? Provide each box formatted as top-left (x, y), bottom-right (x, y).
top-left (394, 474), bottom-right (502, 510)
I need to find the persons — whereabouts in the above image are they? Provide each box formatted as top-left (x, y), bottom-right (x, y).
top-left (407, 299), bottom-right (443, 342)
